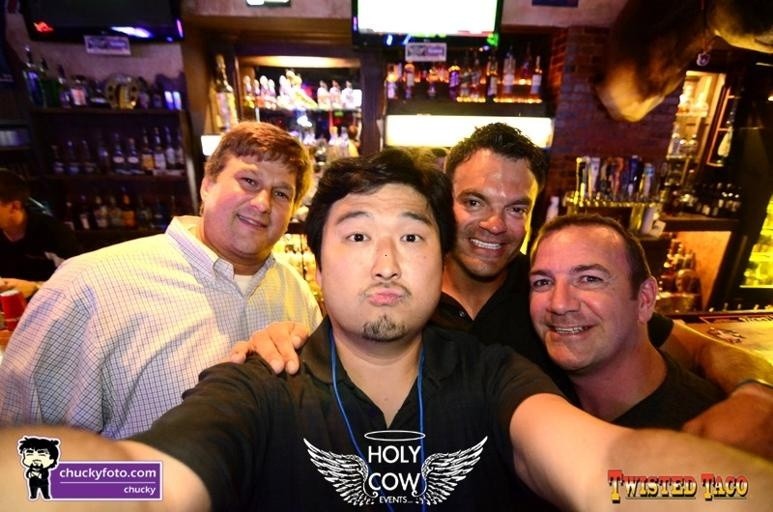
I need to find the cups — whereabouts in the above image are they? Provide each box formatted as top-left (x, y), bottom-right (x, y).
top-left (1, 288), bottom-right (26, 332)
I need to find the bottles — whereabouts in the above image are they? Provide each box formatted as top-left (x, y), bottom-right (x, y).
top-left (38, 123), bottom-right (185, 176)
top-left (572, 152), bottom-right (654, 203)
top-left (244, 70), bottom-right (362, 165)
top-left (267, 235), bottom-right (324, 302)
top-left (664, 82), bottom-right (714, 157)
top-left (382, 41), bottom-right (546, 104)
top-left (16, 46), bottom-right (187, 112)
top-left (205, 53), bottom-right (240, 137)
top-left (49, 185), bottom-right (190, 234)
top-left (655, 232), bottom-right (700, 296)
top-left (653, 156), bottom-right (742, 220)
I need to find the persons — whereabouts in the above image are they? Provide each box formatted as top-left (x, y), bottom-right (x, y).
top-left (0, 169), bottom-right (83, 281)
top-left (220, 121), bottom-right (773, 464)
top-left (213, 52), bottom-right (363, 153)
top-left (527, 211), bottom-right (727, 430)
top-left (0, 121), bottom-right (324, 511)
top-left (383, 52), bottom-right (543, 100)
top-left (0, 145), bottom-right (773, 512)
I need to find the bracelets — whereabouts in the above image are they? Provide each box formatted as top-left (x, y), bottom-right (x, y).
top-left (730, 373), bottom-right (773, 393)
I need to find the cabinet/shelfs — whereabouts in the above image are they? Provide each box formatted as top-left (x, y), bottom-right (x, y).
top-left (27, 100), bottom-right (190, 242)
